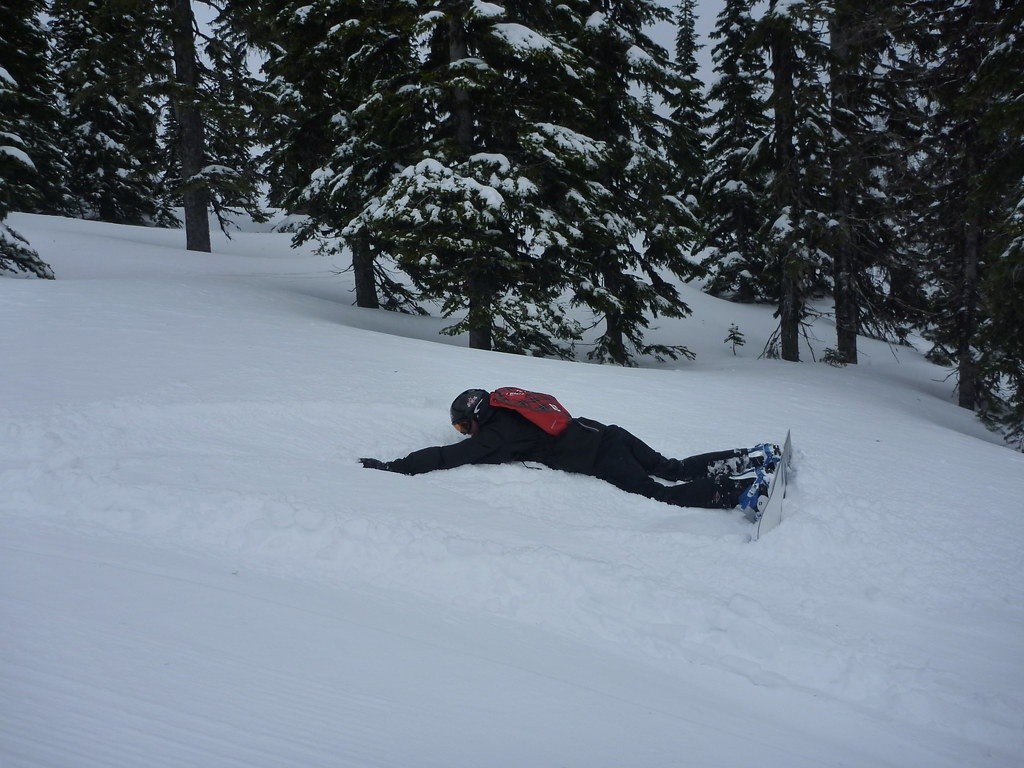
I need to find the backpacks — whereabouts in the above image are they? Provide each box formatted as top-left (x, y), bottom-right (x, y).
top-left (490, 387), bottom-right (572, 435)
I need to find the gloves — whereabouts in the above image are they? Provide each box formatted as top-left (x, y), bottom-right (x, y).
top-left (359, 458), bottom-right (386, 470)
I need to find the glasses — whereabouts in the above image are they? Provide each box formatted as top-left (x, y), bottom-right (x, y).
top-left (461, 421), bottom-right (470, 434)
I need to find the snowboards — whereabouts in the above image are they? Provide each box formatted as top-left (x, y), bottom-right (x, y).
top-left (756, 427), bottom-right (793, 540)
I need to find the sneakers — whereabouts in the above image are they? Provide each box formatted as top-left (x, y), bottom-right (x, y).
top-left (734, 443), bottom-right (780, 470)
top-left (728, 466), bottom-right (773, 516)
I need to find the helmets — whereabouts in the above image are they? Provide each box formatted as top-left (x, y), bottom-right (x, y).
top-left (450, 389), bottom-right (490, 424)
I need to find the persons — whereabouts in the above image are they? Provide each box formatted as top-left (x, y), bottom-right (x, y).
top-left (354, 389), bottom-right (782, 521)
